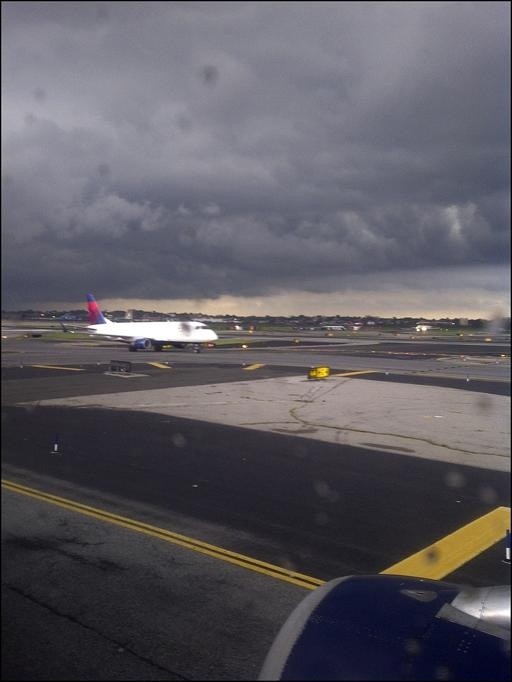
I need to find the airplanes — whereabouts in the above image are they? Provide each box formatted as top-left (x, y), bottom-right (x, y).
top-left (61, 293), bottom-right (219, 353)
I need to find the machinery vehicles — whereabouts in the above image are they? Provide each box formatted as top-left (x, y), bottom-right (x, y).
top-left (308, 367), bottom-right (330, 379)
